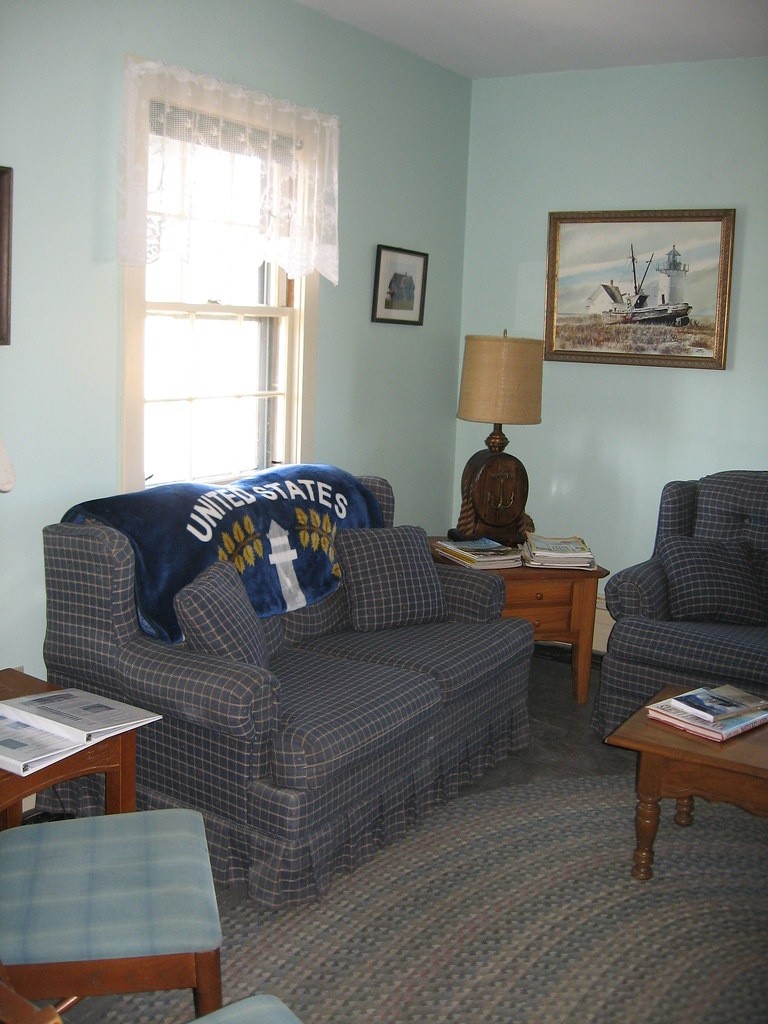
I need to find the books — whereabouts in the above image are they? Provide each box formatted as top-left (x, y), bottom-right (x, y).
top-left (645, 684), bottom-right (768, 743)
top-left (434, 530), bottom-right (598, 571)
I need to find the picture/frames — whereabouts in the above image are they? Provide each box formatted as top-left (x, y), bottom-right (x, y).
top-left (371, 245), bottom-right (429, 326)
top-left (544, 209), bottom-right (737, 370)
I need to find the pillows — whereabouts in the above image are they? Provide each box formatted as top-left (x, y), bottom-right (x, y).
top-left (174, 562), bottom-right (269, 668)
top-left (656, 536), bottom-right (765, 626)
top-left (335, 526), bottom-right (448, 633)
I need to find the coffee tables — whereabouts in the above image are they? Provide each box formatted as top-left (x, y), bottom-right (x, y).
top-left (604, 686), bottom-right (768, 880)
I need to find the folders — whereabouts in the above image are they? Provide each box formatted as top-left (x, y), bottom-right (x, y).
top-left (0, 688), bottom-right (164, 777)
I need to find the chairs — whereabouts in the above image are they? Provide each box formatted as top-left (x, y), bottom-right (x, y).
top-left (0, 808), bottom-right (224, 1018)
top-left (0, 977), bottom-right (304, 1024)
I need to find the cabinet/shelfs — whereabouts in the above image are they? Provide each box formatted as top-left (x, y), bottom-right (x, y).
top-left (426, 535), bottom-right (610, 703)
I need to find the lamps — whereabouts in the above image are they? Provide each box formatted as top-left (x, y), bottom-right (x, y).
top-left (447, 329), bottom-right (546, 548)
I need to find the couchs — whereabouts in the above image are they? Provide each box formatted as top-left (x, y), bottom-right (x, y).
top-left (43, 464), bottom-right (534, 907)
top-left (590, 467), bottom-right (768, 741)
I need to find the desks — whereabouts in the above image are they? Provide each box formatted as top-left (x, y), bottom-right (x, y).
top-left (0, 668), bottom-right (136, 831)
